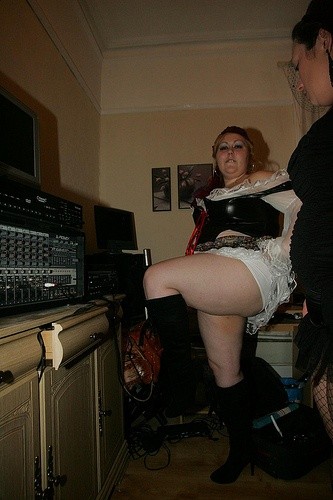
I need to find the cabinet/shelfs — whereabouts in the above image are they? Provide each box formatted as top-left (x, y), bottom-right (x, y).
top-left (0, 294), bottom-right (129, 500)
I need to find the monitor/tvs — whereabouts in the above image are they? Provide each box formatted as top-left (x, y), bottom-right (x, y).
top-left (94, 205), bottom-right (138, 254)
top-left (0, 85), bottom-right (41, 189)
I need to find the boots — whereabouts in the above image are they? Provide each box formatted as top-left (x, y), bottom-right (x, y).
top-left (145, 293), bottom-right (210, 416)
top-left (210, 377), bottom-right (257, 484)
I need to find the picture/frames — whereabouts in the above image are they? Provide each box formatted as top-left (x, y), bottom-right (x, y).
top-left (177, 163), bottom-right (214, 209)
top-left (151, 167), bottom-right (172, 212)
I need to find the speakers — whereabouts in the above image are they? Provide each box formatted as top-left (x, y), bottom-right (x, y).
top-left (246, 404), bottom-right (333, 480)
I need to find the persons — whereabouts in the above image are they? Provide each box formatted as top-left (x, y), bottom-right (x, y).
top-left (143, 126), bottom-right (303, 484)
top-left (286, 0), bottom-right (333, 440)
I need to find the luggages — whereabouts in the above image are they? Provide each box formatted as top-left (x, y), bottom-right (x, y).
top-left (250, 356), bottom-right (331, 482)
